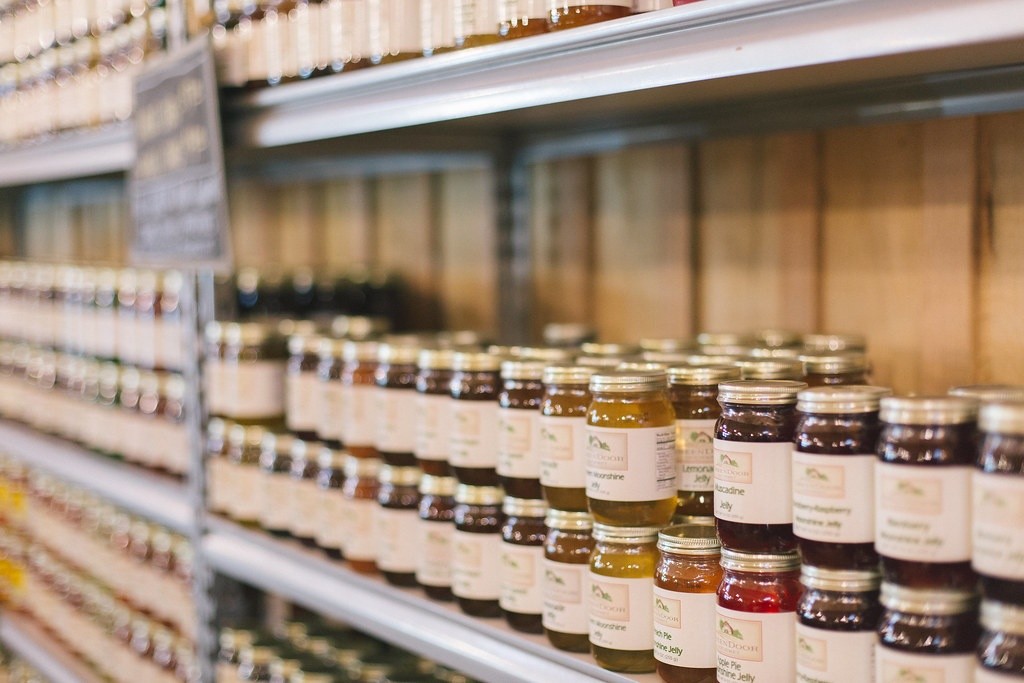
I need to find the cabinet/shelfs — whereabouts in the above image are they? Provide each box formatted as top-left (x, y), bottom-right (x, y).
top-left (0, 0), bottom-right (1024, 683)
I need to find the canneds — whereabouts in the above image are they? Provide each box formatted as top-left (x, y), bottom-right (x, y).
top-left (0, 0), bottom-right (1024, 683)
